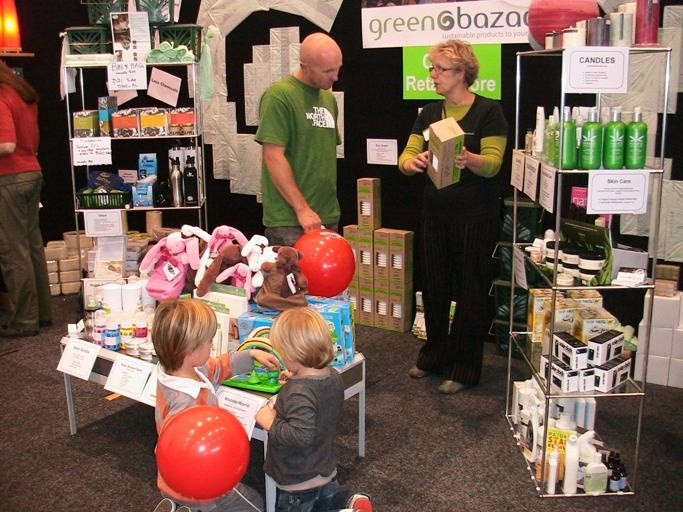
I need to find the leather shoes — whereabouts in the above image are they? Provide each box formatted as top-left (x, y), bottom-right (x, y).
top-left (408, 364), bottom-right (432, 379)
top-left (437, 379), bottom-right (465, 394)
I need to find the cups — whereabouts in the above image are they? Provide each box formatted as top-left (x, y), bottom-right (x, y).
top-left (544, 240), bottom-right (605, 280)
top-left (543, 0), bottom-right (660, 48)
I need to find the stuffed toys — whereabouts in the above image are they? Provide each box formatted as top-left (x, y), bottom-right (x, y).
top-left (138, 225), bottom-right (308, 312)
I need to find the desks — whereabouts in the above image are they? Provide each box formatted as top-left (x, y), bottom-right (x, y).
top-left (57, 332), bottom-right (367, 512)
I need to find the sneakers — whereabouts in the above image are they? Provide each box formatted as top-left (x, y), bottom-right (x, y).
top-left (0, 324), bottom-right (40, 339)
top-left (38, 319), bottom-right (53, 329)
top-left (348, 494), bottom-right (373, 512)
top-left (153, 498), bottom-right (192, 512)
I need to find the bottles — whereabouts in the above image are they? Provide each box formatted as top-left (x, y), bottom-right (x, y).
top-left (524, 105), bottom-right (647, 171)
top-left (605, 448), bottom-right (628, 493)
top-left (94, 308), bottom-right (155, 352)
top-left (536, 412), bottom-right (608, 495)
top-left (153, 156), bottom-right (197, 208)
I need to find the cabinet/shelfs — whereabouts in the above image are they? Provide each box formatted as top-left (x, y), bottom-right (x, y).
top-left (61, 60), bottom-right (209, 308)
top-left (501, 46), bottom-right (674, 501)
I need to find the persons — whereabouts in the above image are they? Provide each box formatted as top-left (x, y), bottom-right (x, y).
top-left (396, 40), bottom-right (512, 392)
top-left (0, 59), bottom-right (52, 337)
top-left (252, 33), bottom-right (343, 250)
top-left (150, 298), bottom-right (281, 511)
top-left (254, 307), bottom-right (373, 512)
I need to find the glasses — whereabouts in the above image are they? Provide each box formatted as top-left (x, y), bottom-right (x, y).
top-left (429, 65), bottom-right (452, 73)
